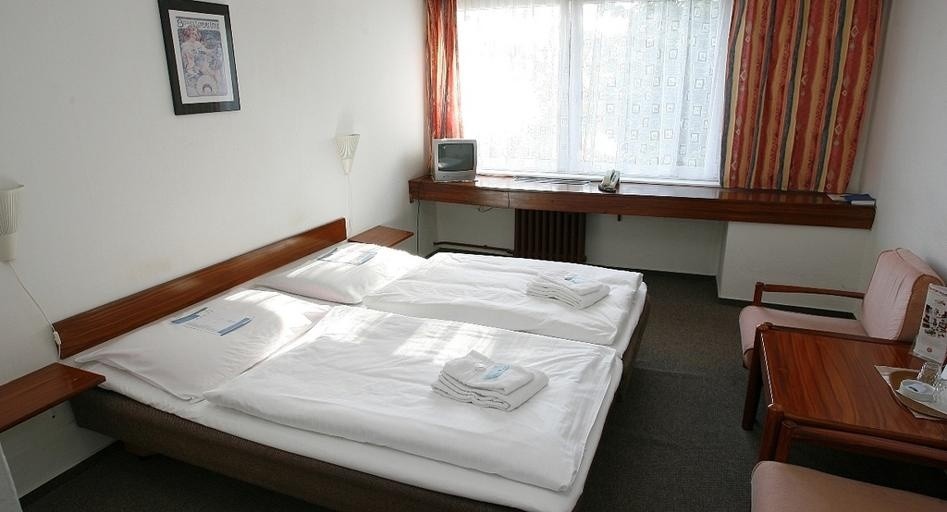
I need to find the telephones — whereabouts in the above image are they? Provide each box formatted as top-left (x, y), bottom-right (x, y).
top-left (601, 169), bottom-right (620, 189)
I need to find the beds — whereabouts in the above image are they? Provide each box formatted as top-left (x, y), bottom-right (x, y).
top-left (241, 239), bottom-right (651, 369)
top-left (67, 289), bottom-right (625, 511)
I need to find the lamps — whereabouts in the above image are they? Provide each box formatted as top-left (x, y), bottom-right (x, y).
top-left (333, 134), bottom-right (358, 175)
top-left (0, 182), bottom-right (25, 262)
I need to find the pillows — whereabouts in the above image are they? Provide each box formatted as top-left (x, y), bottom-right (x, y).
top-left (76, 287), bottom-right (332, 405)
top-left (253, 240), bottom-right (426, 304)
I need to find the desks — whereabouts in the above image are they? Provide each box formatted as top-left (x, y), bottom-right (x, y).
top-left (741, 322), bottom-right (947, 469)
top-left (348, 224), bottom-right (415, 247)
top-left (0, 363), bottom-right (106, 436)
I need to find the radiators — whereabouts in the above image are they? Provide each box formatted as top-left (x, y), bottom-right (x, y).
top-left (513, 208), bottom-right (587, 264)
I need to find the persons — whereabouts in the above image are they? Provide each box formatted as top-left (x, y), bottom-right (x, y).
top-left (181, 25), bottom-right (218, 76)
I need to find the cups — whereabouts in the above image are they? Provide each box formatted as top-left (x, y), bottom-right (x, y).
top-left (917, 361), bottom-right (947, 413)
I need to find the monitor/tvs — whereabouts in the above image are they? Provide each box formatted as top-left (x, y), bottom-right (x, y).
top-left (432, 138), bottom-right (477, 183)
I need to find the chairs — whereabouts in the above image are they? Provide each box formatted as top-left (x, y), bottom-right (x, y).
top-left (737, 248), bottom-right (946, 369)
top-left (750, 460), bottom-right (947, 512)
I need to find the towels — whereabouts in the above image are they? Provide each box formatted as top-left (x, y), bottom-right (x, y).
top-left (432, 349), bottom-right (549, 412)
top-left (526, 270), bottom-right (611, 310)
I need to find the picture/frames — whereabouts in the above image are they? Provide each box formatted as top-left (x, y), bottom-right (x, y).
top-left (157, 0), bottom-right (241, 115)
